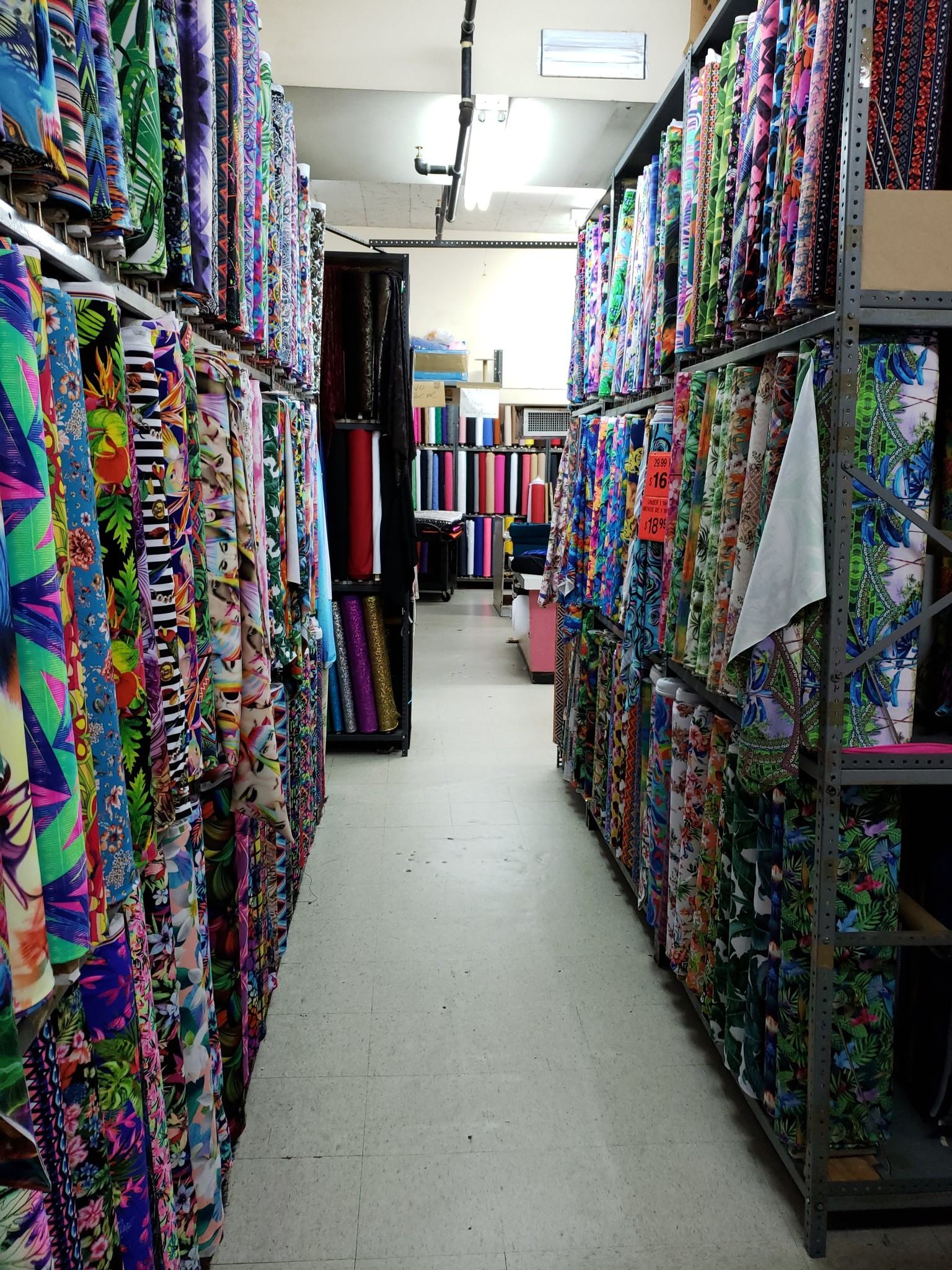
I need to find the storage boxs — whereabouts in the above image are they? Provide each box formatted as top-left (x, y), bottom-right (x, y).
top-left (414, 350), bottom-right (468, 381)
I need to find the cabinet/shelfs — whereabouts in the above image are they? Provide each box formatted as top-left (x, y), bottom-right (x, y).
top-left (0, 0), bottom-right (952, 1270)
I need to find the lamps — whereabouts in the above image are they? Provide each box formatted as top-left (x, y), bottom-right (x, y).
top-left (464, 94), bottom-right (509, 211)
